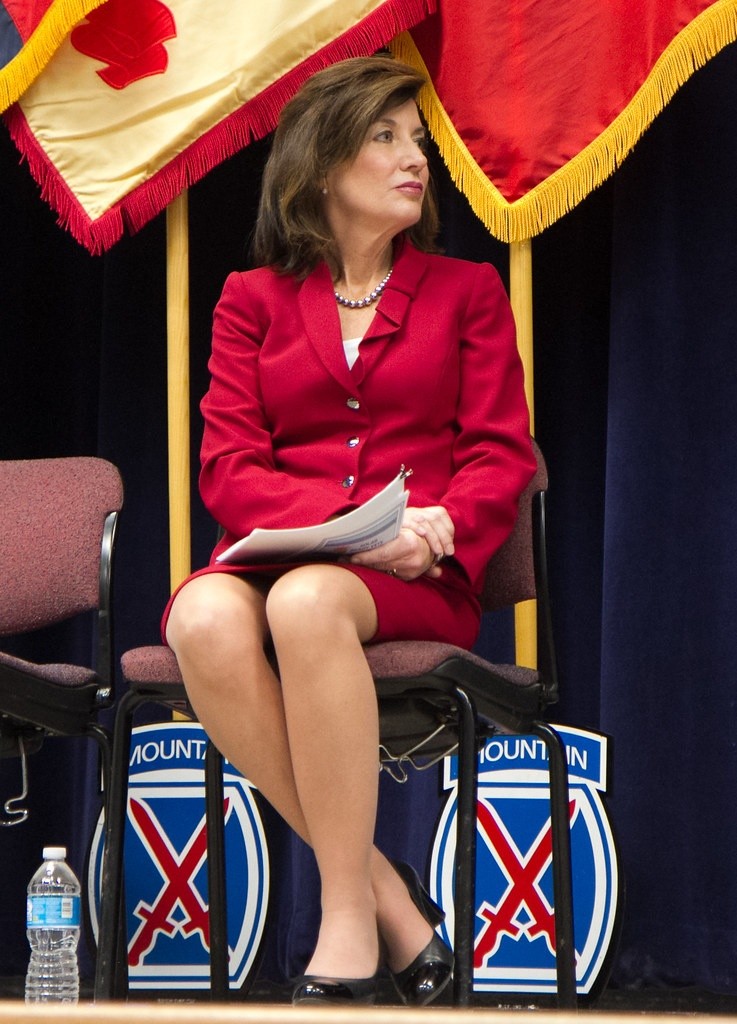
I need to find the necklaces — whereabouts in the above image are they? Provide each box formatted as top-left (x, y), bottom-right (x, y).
top-left (334, 266), bottom-right (394, 307)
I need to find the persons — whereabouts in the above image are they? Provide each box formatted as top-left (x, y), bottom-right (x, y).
top-left (158, 57), bottom-right (539, 1008)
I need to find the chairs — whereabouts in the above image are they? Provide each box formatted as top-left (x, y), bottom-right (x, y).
top-left (93, 434), bottom-right (578, 1011)
top-left (1, 457), bottom-right (129, 1001)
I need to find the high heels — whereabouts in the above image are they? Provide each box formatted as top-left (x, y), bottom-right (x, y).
top-left (380, 857), bottom-right (455, 1008)
top-left (293, 931), bottom-right (386, 1006)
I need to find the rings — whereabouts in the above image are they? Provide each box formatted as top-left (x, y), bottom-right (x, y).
top-left (386, 568), bottom-right (398, 576)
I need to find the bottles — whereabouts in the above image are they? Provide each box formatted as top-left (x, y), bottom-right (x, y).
top-left (24, 848), bottom-right (81, 1005)
